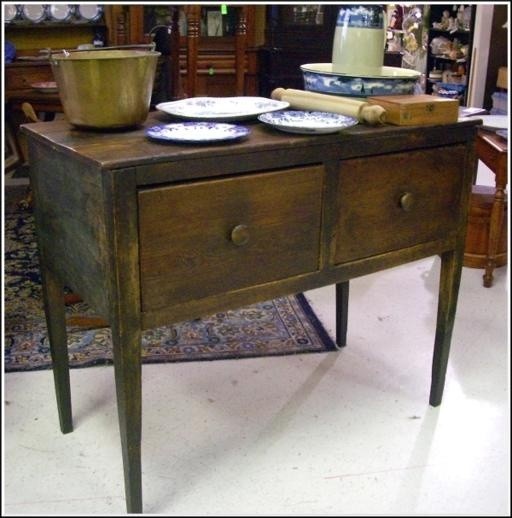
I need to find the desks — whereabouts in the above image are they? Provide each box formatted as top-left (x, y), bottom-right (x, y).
top-left (18, 107), bottom-right (485, 513)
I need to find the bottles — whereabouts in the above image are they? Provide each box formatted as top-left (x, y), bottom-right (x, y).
top-left (332, 6), bottom-right (385, 76)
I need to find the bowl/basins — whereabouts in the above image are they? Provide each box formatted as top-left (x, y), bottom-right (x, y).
top-left (301, 59), bottom-right (421, 96)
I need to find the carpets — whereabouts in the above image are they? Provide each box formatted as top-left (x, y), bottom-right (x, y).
top-left (5, 182), bottom-right (341, 375)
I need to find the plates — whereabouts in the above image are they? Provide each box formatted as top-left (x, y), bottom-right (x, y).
top-left (147, 95), bottom-right (358, 146)
top-left (4, 4), bottom-right (102, 23)
top-left (30, 82), bottom-right (58, 93)
